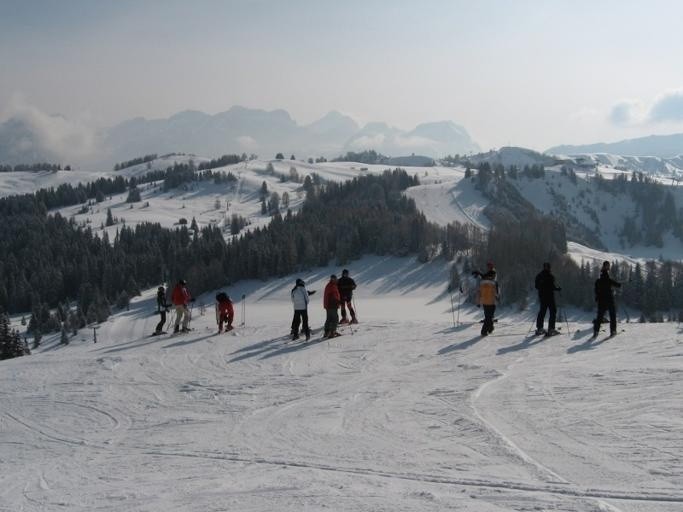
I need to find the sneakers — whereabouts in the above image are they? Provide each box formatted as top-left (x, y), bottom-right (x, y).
top-left (291, 318), bottom-right (358, 340)
top-left (610, 331), bottom-right (617, 336)
top-left (535, 328), bottom-right (560, 336)
top-left (217, 326), bottom-right (233, 334)
top-left (155, 328), bottom-right (191, 335)
top-left (593, 331), bottom-right (599, 336)
top-left (481, 327), bottom-right (495, 336)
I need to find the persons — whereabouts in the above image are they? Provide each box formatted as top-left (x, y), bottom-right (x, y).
top-left (338, 269), bottom-right (359, 324)
top-left (472, 262), bottom-right (498, 282)
top-left (155, 287), bottom-right (171, 334)
top-left (213, 291), bottom-right (233, 332)
top-left (291, 281), bottom-right (313, 339)
top-left (592, 272), bottom-right (622, 337)
top-left (322, 274), bottom-right (341, 339)
top-left (598, 261), bottom-right (610, 323)
top-left (289, 278), bottom-right (316, 334)
top-left (533, 262), bottom-right (562, 335)
top-left (477, 271), bottom-right (502, 335)
top-left (171, 280), bottom-right (196, 333)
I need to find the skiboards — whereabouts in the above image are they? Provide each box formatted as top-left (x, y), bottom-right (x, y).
top-left (145, 332), bottom-right (168, 337)
top-left (284, 332), bottom-right (315, 345)
top-left (525, 332), bottom-right (561, 339)
top-left (211, 323), bottom-right (246, 335)
top-left (318, 334), bottom-right (354, 342)
top-left (588, 329), bottom-right (625, 346)
top-left (170, 328), bottom-right (197, 336)
